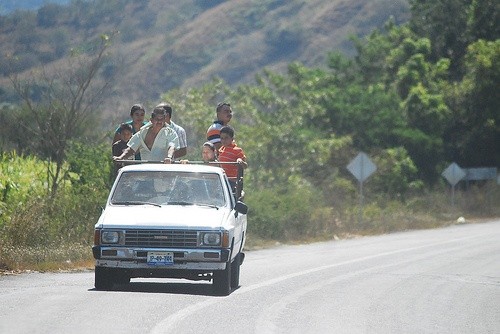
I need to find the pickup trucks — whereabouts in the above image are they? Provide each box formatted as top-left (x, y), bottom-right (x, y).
top-left (91, 160), bottom-right (247, 296)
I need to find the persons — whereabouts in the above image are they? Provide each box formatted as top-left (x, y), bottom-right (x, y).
top-left (112, 103), bottom-right (187, 169)
top-left (173, 183), bottom-right (192, 200)
top-left (180, 142), bottom-right (219, 164)
top-left (206, 102), bottom-right (247, 192)
